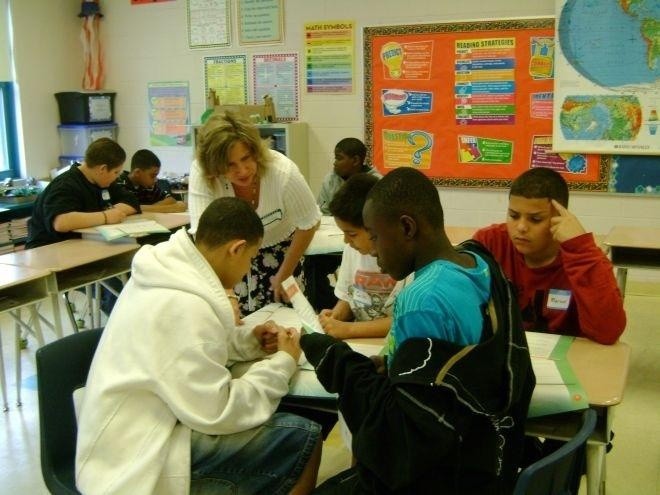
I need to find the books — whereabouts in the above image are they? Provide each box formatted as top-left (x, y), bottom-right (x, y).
top-left (0, 216), bottom-right (33, 255)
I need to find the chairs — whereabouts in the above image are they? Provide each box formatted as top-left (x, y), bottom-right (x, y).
top-left (36, 326), bottom-right (106, 495)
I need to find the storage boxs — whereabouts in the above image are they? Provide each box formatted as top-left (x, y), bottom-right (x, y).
top-left (53, 89), bottom-right (118, 170)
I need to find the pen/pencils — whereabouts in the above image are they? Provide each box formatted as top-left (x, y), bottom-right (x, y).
top-left (108, 202), bottom-right (114, 208)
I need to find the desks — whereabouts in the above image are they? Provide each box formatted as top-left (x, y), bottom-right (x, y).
top-left (230, 325), bottom-right (633, 492)
top-left (0, 212), bottom-right (190, 413)
top-left (297, 215), bottom-right (660, 311)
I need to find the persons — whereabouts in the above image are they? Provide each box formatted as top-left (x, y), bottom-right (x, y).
top-left (473, 167), bottom-right (626, 345)
top-left (318, 174), bottom-right (414, 339)
top-left (299, 168), bottom-right (536, 495)
top-left (188, 109), bottom-right (321, 317)
top-left (25, 138), bottom-right (142, 316)
top-left (317, 138), bottom-right (383, 216)
top-left (73, 199), bottom-right (322, 495)
